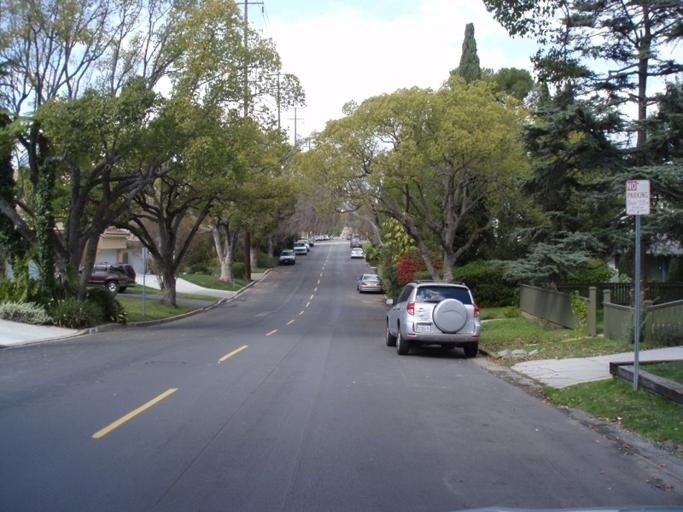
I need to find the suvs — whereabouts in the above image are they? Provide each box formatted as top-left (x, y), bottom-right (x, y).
top-left (78, 263), bottom-right (136, 293)
top-left (384, 281), bottom-right (481, 355)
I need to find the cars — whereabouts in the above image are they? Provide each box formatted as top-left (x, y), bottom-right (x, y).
top-left (280, 248), bottom-right (294, 264)
top-left (346, 233), bottom-right (362, 247)
top-left (351, 248), bottom-right (363, 258)
top-left (357, 273), bottom-right (383, 293)
top-left (294, 238), bottom-right (313, 255)
